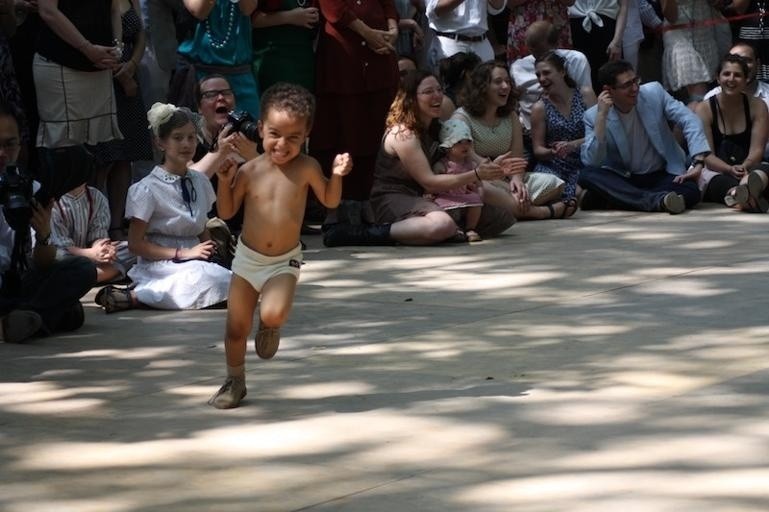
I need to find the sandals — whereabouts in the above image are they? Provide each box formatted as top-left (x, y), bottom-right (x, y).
top-left (539, 197), bottom-right (577, 217)
top-left (104, 287), bottom-right (133, 313)
top-left (94, 285), bottom-right (136, 305)
top-left (746, 172), bottom-right (768, 212)
top-left (724, 185), bottom-right (749, 206)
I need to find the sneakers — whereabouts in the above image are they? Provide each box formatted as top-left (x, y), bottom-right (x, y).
top-left (208, 373), bottom-right (247, 409)
top-left (662, 192), bottom-right (686, 213)
top-left (256, 318), bottom-right (280, 359)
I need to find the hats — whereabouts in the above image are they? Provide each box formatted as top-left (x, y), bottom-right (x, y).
top-left (439, 119), bottom-right (474, 152)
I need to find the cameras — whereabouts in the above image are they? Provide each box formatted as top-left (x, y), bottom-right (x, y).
top-left (0, 164), bottom-right (35, 228)
top-left (221, 109), bottom-right (263, 148)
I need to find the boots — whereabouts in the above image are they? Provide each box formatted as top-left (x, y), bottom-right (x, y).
top-left (323, 222), bottom-right (395, 246)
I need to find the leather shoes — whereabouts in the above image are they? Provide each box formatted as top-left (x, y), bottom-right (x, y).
top-left (1, 311), bottom-right (41, 342)
top-left (466, 229), bottom-right (480, 241)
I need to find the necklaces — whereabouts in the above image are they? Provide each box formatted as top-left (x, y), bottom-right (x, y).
top-left (203, 2), bottom-right (235, 49)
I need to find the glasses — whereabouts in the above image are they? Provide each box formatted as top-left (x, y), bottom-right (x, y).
top-left (616, 77), bottom-right (640, 89)
top-left (416, 87), bottom-right (444, 94)
top-left (202, 89), bottom-right (232, 98)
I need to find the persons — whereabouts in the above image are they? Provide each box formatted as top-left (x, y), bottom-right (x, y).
top-left (576, 60), bottom-right (712, 217)
top-left (716, 1), bottom-right (768, 85)
top-left (703, 43), bottom-right (769, 112)
top-left (448, 61), bottom-right (578, 220)
top-left (31, 0), bottom-right (124, 212)
top-left (622, 1), bottom-right (663, 77)
top-left (505, 1), bottom-right (575, 65)
top-left (94, 103), bottom-right (235, 314)
top-left (166, 1), bottom-right (264, 133)
top-left (426, 0), bottom-right (508, 82)
top-left (251, 1), bottom-right (322, 98)
top-left (529, 52), bottom-right (598, 201)
top-left (324, 70), bottom-right (529, 248)
top-left (182, 76), bottom-right (265, 276)
top-left (134, 0), bottom-right (175, 114)
top-left (394, 0), bottom-right (426, 58)
top-left (693, 53), bottom-right (769, 212)
top-left (421, 119), bottom-right (484, 240)
top-left (206, 86), bottom-right (353, 409)
top-left (567, 1), bottom-right (630, 63)
top-left (314, 1), bottom-right (401, 224)
top-left (47, 181), bottom-right (137, 288)
top-left (114, 0), bottom-right (149, 167)
top-left (659, 2), bottom-right (734, 146)
top-left (396, 57), bottom-right (420, 88)
top-left (0, 0), bottom-right (35, 174)
top-left (0, 113), bottom-right (97, 342)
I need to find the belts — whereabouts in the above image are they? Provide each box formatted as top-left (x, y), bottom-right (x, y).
top-left (436, 31), bottom-right (489, 42)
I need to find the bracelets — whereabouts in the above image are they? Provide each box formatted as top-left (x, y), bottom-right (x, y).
top-left (78, 39), bottom-right (90, 52)
top-left (113, 39), bottom-right (125, 58)
top-left (35, 230), bottom-right (53, 246)
top-left (691, 160), bottom-right (704, 168)
top-left (473, 168), bottom-right (484, 183)
top-left (612, 41), bottom-right (623, 48)
top-left (171, 246), bottom-right (181, 262)
top-left (387, 25), bottom-right (399, 30)
top-left (130, 57), bottom-right (140, 68)
